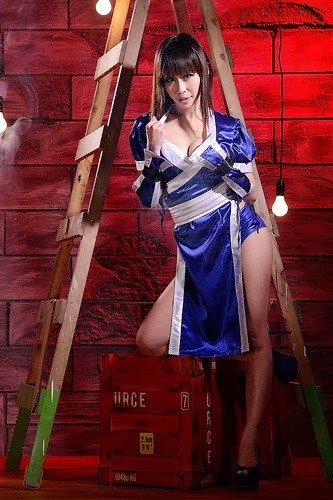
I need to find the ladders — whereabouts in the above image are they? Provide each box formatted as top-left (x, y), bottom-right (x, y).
top-left (5, 0), bottom-right (333, 490)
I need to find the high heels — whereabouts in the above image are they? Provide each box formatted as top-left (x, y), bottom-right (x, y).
top-left (268, 331), bottom-right (298, 384)
top-left (235, 445), bottom-right (261, 482)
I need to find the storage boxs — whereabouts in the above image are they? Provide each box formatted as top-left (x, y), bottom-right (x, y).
top-left (96, 350), bottom-right (291, 488)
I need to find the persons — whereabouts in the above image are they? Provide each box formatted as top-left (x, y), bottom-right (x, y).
top-left (130, 33), bottom-right (273, 489)
